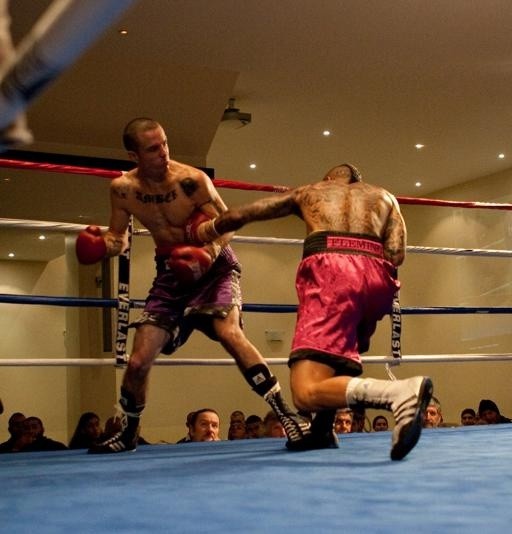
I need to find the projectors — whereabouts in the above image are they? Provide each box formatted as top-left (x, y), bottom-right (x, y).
top-left (218, 113), bottom-right (251, 131)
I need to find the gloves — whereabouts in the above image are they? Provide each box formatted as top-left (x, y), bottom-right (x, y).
top-left (182, 209), bottom-right (212, 244)
top-left (75, 224), bottom-right (106, 265)
top-left (383, 261), bottom-right (400, 292)
top-left (167, 246), bottom-right (214, 283)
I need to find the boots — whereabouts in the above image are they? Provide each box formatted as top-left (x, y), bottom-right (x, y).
top-left (350, 376), bottom-right (434, 463)
top-left (285, 409), bottom-right (339, 452)
top-left (86, 387), bottom-right (148, 455)
top-left (251, 375), bottom-right (303, 442)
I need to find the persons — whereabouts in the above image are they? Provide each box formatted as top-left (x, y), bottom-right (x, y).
top-left (373, 415), bottom-right (388, 432)
top-left (0, 398), bottom-right (152, 453)
top-left (333, 408), bottom-right (354, 434)
top-left (423, 397), bottom-right (444, 427)
top-left (295, 408), bottom-right (312, 422)
top-left (177, 408), bottom-right (221, 443)
top-left (227, 411), bottom-right (287, 440)
top-left (76, 117), bottom-right (311, 452)
top-left (182, 164), bottom-right (433, 460)
top-left (461, 399), bottom-right (511, 426)
